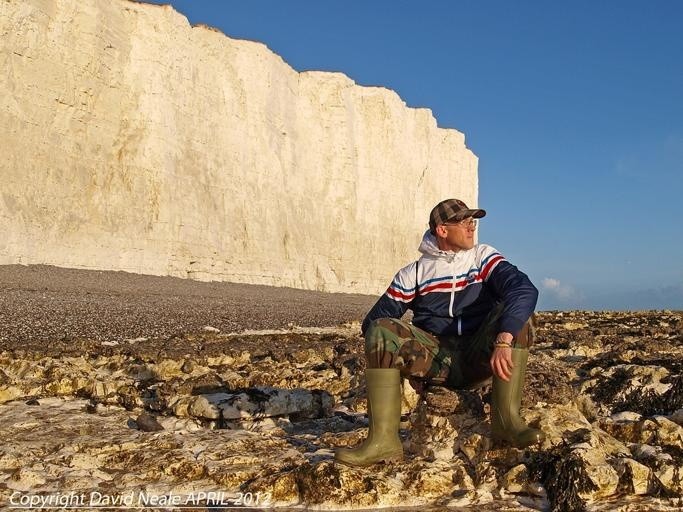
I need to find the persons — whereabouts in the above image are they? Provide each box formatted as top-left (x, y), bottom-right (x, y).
top-left (334, 197), bottom-right (546, 468)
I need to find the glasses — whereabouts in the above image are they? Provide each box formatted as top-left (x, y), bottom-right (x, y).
top-left (443, 220), bottom-right (478, 228)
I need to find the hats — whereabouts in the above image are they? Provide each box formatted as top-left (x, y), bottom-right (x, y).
top-left (430, 199), bottom-right (486, 232)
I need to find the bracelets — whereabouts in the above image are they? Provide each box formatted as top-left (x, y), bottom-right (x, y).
top-left (494, 340), bottom-right (511, 348)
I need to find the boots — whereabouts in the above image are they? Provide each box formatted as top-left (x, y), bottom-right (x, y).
top-left (489, 347), bottom-right (545, 448)
top-left (335, 368), bottom-right (404, 465)
